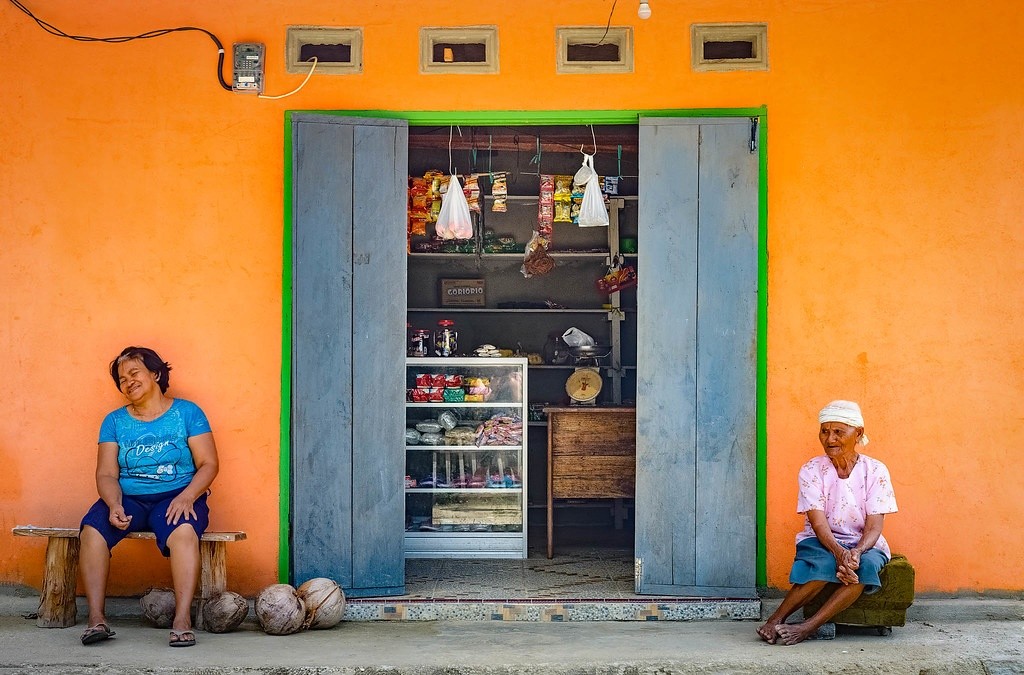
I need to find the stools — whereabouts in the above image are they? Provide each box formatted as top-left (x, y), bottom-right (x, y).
top-left (803, 554), bottom-right (915, 640)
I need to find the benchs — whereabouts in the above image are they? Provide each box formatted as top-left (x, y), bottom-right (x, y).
top-left (13, 527), bottom-right (247, 629)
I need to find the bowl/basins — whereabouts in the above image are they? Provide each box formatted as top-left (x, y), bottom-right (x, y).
top-left (565, 345), bottom-right (613, 358)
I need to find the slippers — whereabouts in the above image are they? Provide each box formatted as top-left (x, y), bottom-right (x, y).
top-left (80, 623), bottom-right (116, 645)
top-left (169, 629), bottom-right (197, 646)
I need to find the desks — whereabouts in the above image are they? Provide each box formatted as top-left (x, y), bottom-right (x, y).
top-left (542, 407), bottom-right (635, 560)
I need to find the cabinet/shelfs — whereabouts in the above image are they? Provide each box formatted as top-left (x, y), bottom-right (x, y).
top-left (407, 358), bottom-right (529, 559)
top-left (408, 193), bottom-right (637, 528)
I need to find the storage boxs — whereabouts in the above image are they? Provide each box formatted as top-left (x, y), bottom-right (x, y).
top-left (439, 279), bottom-right (486, 309)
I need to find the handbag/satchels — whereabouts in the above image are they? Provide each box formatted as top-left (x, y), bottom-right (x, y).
top-left (578, 156), bottom-right (609, 227)
top-left (435, 175), bottom-right (472, 240)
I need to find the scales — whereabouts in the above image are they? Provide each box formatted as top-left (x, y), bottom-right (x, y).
top-left (562, 343), bottom-right (616, 407)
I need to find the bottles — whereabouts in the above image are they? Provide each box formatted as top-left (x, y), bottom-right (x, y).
top-left (543, 335), bottom-right (569, 365)
top-left (411, 329), bottom-right (431, 357)
top-left (433, 320), bottom-right (458, 356)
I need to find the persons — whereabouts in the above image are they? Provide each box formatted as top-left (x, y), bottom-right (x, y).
top-left (77, 344), bottom-right (221, 648)
top-left (755, 399), bottom-right (901, 647)
